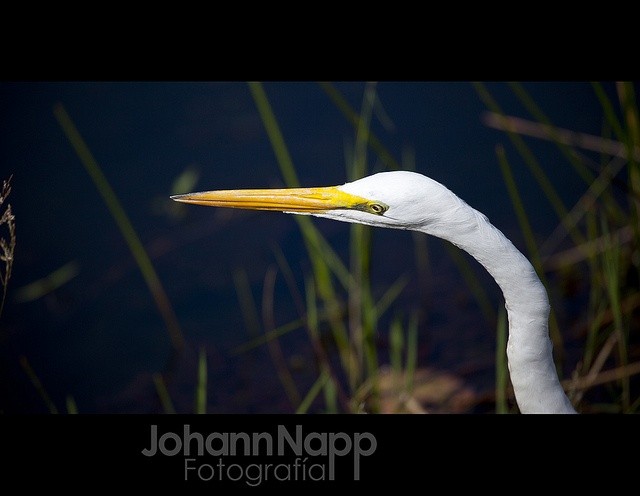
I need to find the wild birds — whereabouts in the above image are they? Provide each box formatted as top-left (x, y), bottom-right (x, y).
top-left (168, 170), bottom-right (579, 415)
